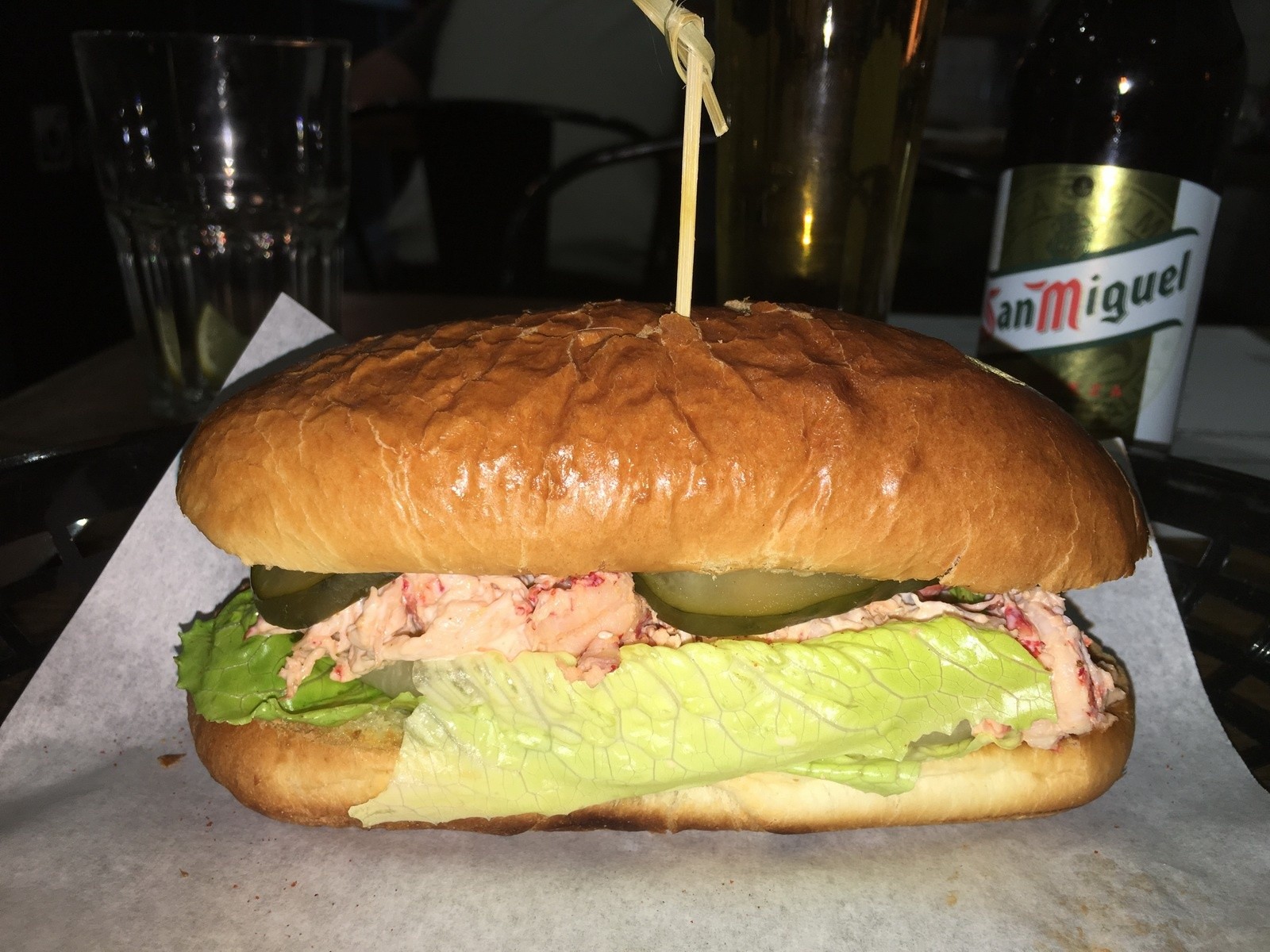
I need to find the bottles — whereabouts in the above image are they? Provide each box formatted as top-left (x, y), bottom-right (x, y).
top-left (978, 1), bottom-right (1249, 518)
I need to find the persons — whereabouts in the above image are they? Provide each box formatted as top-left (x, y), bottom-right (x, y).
top-left (352, 0), bottom-right (680, 283)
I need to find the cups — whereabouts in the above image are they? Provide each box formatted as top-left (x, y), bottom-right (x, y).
top-left (710, 0), bottom-right (946, 323)
top-left (68, 26), bottom-right (352, 424)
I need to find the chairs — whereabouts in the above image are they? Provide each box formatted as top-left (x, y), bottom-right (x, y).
top-left (345, 91), bottom-right (998, 313)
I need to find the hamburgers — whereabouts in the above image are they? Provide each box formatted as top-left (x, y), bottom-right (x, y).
top-left (173, 300), bottom-right (1147, 836)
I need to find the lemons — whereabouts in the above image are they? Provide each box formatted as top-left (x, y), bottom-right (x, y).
top-left (158, 301), bottom-right (251, 381)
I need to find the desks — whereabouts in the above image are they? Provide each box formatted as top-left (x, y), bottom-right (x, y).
top-left (0, 289), bottom-right (1269, 790)
